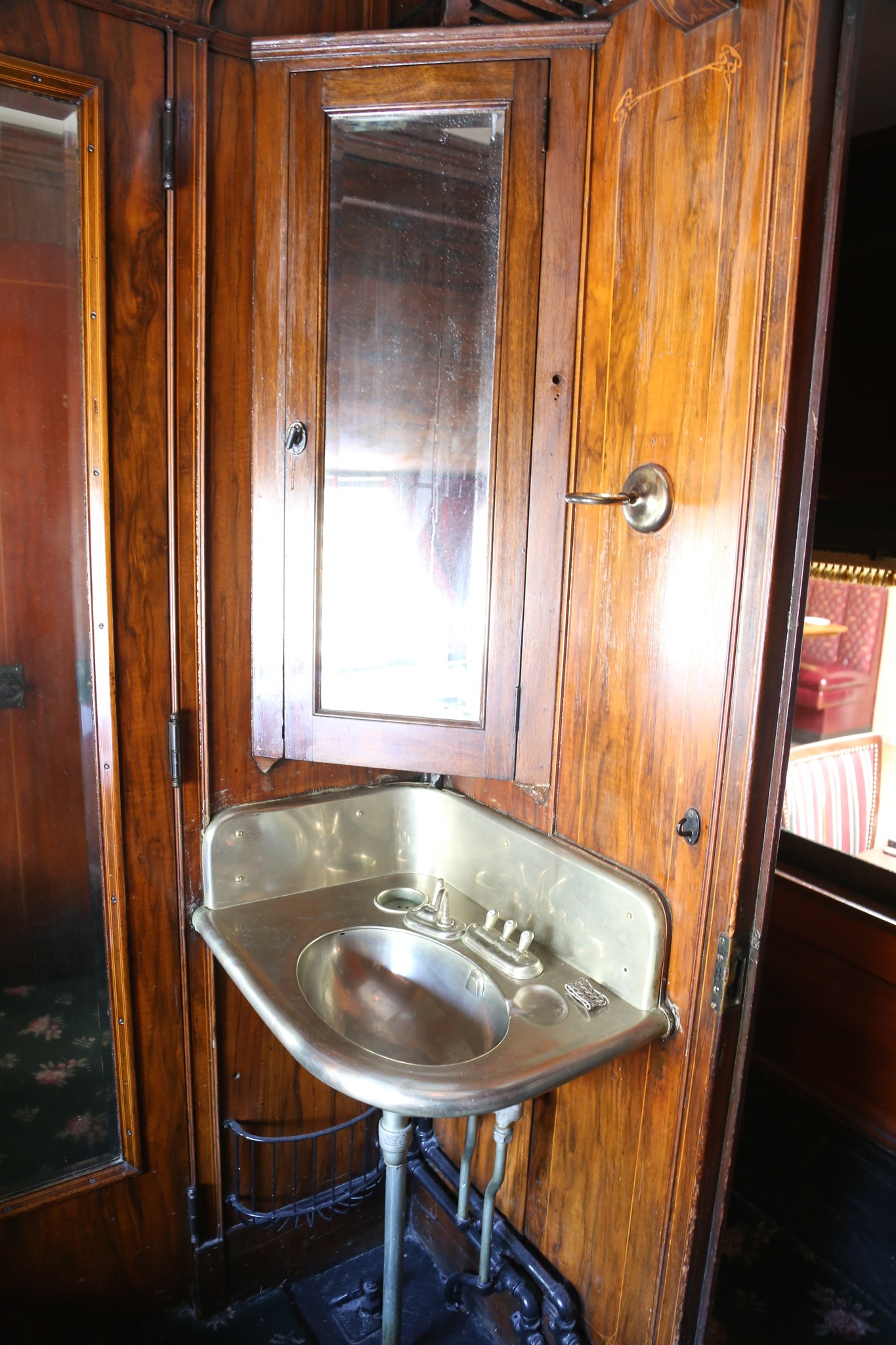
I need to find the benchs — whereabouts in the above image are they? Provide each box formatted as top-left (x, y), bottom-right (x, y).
top-left (797, 579), bottom-right (892, 746)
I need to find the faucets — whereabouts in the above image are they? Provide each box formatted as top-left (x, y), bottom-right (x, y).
top-left (403, 878), bottom-right (465, 942)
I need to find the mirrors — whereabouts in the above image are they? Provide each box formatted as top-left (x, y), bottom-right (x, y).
top-left (292, 69), bottom-right (530, 774)
top-left (0, 47), bottom-right (144, 1221)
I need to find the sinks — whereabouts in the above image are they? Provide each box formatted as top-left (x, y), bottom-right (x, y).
top-left (293, 922), bottom-right (512, 1069)
